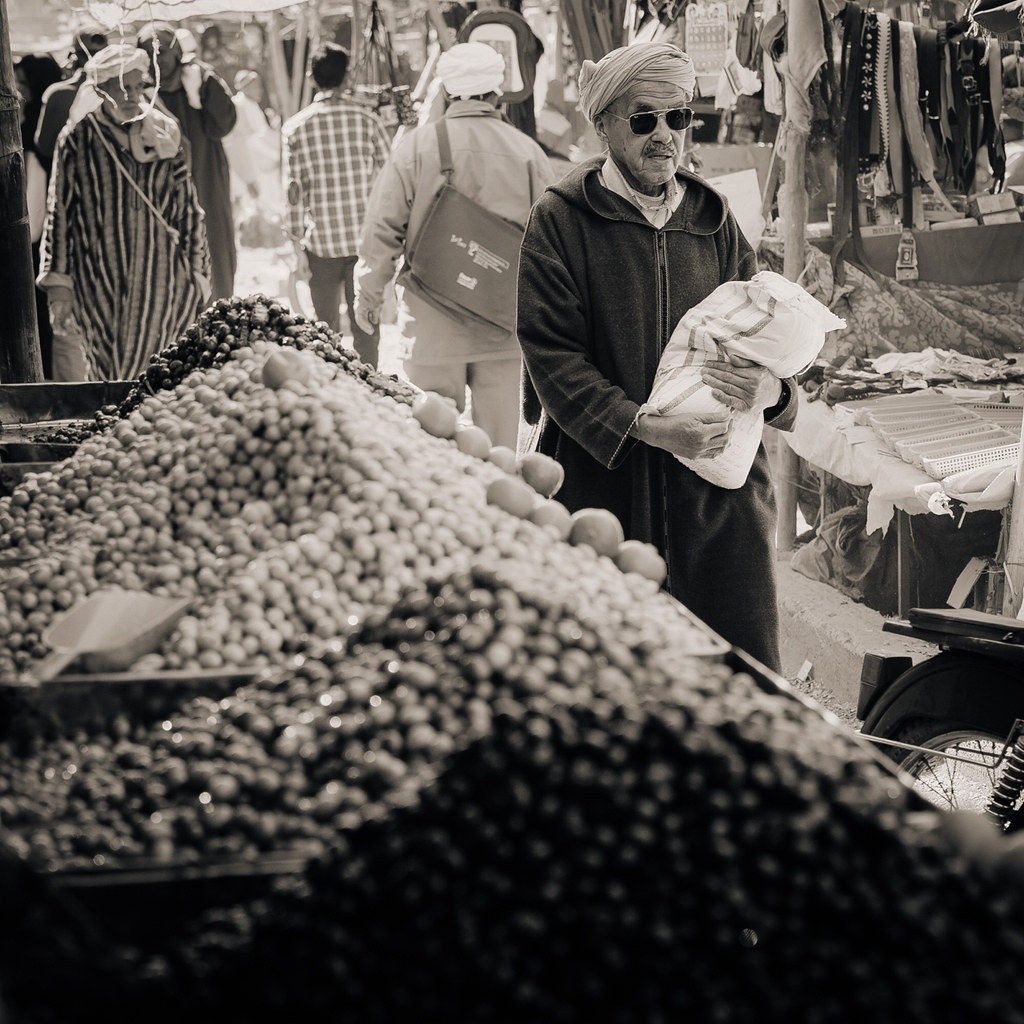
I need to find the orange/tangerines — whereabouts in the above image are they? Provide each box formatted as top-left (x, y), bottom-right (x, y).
top-left (414, 392), bottom-right (667, 589)
top-left (263, 349), bottom-right (309, 386)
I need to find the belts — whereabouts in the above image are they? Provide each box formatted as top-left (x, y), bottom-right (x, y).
top-left (829, 2), bottom-right (1007, 287)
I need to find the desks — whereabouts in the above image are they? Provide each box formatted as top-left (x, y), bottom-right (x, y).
top-left (762, 346), bottom-right (1024, 621)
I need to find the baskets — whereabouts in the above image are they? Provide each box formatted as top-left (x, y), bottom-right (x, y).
top-left (833, 395), bottom-right (1024, 480)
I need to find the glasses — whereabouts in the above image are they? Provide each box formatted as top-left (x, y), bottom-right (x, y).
top-left (602, 107), bottom-right (694, 136)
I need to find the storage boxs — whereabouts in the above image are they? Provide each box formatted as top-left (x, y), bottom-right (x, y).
top-left (805, 188), bottom-right (1022, 243)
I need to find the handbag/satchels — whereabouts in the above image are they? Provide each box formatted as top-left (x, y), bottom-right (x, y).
top-left (344, 84), bottom-right (419, 126)
top-left (395, 184), bottom-right (526, 343)
top-left (791, 505), bottom-right (888, 603)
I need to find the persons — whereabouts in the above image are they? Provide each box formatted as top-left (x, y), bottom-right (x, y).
top-left (12, 21), bottom-right (280, 385)
top-left (282, 40), bottom-right (390, 375)
top-left (515, 39), bottom-right (799, 691)
top-left (354, 44), bottom-right (556, 457)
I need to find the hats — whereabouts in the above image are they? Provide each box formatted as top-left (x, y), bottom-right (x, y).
top-left (234, 70), bottom-right (258, 90)
top-left (84, 44), bottom-right (151, 87)
top-left (578, 42), bottom-right (696, 121)
top-left (136, 23), bottom-right (182, 60)
top-left (436, 43), bottom-right (506, 101)
